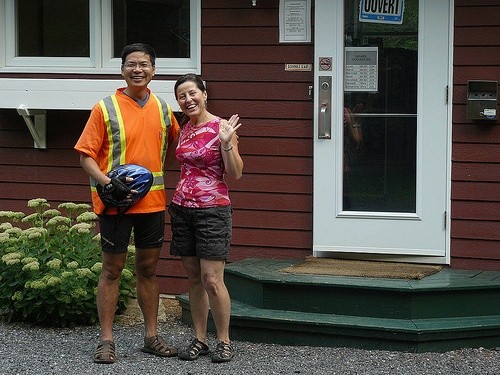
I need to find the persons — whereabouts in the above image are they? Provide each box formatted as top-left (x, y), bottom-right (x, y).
top-left (172, 73), bottom-right (242, 360)
top-left (74, 42), bottom-right (240, 364)
top-left (342, 99), bottom-right (364, 212)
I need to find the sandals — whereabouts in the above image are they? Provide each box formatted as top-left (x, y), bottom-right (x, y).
top-left (94, 340), bottom-right (115, 364)
top-left (140, 336), bottom-right (178, 357)
top-left (178, 338), bottom-right (211, 360)
top-left (211, 341), bottom-right (236, 362)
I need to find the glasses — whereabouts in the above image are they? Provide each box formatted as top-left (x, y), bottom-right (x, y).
top-left (122, 60), bottom-right (154, 70)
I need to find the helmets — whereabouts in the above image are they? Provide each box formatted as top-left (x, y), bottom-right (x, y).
top-left (96, 164), bottom-right (153, 208)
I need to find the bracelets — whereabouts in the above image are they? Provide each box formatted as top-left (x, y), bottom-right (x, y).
top-left (220, 144), bottom-right (234, 152)
